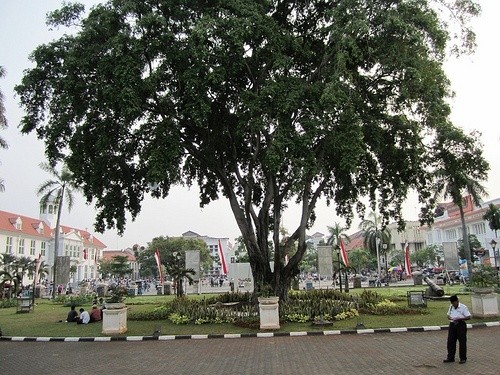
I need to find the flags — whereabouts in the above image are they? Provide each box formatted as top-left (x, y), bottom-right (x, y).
top-left (34, 253), bottom-right (43, 289)
top-left (154, 248), bottom-right (163, 283)
top-left (339, 237), bottom-right (349, 267)
top-left (218, 238), bottom-right (228, 275)
top-left (404, 239), bottom-right (412, 276)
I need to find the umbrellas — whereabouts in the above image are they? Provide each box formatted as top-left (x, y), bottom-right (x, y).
top-left (388, 267), bottom-right (402, 279)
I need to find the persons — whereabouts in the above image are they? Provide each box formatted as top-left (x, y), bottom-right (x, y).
top-left (387, 270), bottom-right (409, 281)
top-left (67, 297), bottom-right (107, 324)
top-left (45, 279), bottom-right (72, 295)
top-left (443, 295), bottom-right (471, 363)
top-left (295, 272), bottom-right (323, 283)
top-left (210, 276), bottom-right (236, 292)
top-left (332, 277), bottom-right (339, 288)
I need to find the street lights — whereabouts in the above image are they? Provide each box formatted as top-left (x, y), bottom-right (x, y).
top-left (382, 240), bottom-right (390, 286)
top-left (334, 245), bottom-right (342, 293)
top-left (489, 239), bottom-right (497, 269)
top-left (176, 252), bottom-right (182, 297)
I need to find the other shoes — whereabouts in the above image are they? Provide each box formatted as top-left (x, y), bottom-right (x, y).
top-left (443, 359), bottom-right (455, 363)
top-left (459, 359), bottom-right (467, 363)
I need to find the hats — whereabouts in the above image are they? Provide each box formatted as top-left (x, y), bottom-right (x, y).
top-left (449, 295), bottom-right (458, 304)
top-left (92, 304), bottom-right (97, 307)
